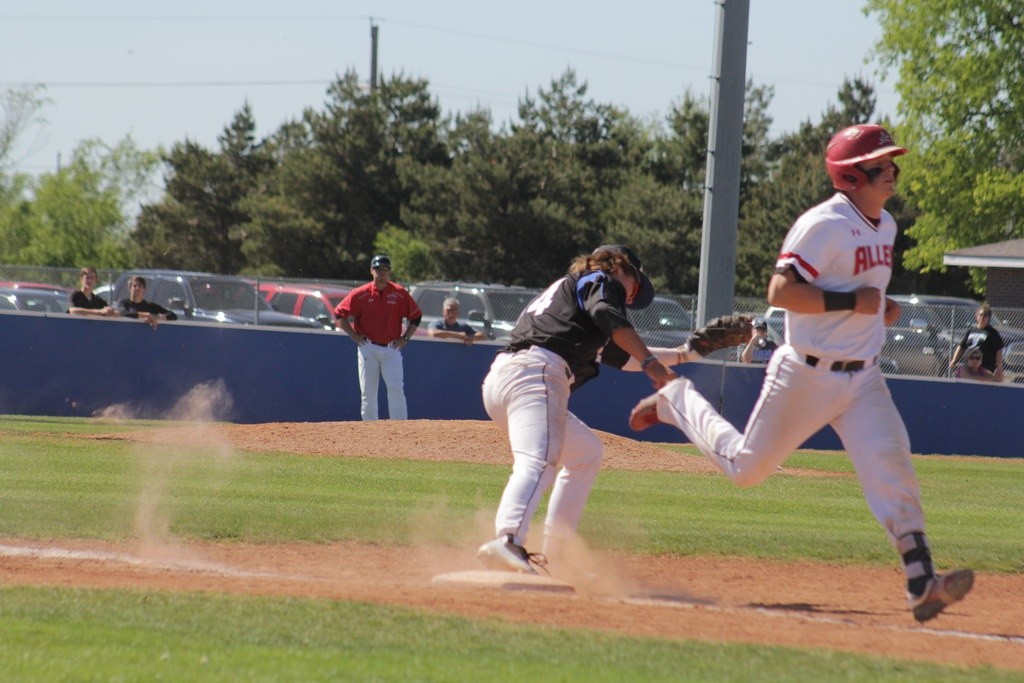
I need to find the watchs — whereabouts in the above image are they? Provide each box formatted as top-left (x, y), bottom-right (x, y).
top-left (402, 334), bottom-right (410, 342)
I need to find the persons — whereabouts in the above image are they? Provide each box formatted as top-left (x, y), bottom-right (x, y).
top-left (476, 244), bottom-right (754, 574)
top-left (736, 317), bottom-right (779, 363)
top-left (65, 266), bottom-right (115, 319)
top-left (427, 297), bottom-right (485, 345)
top-left (333, 256), bottom-right (422, 421)
top-left (627, 124), bottom-right (974, 623)
top-left (116, 276), bottom-right (179, 326)
top-left (947, 306), bottom-right (1008, 384)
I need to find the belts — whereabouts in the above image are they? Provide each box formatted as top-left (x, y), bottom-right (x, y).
top-left (365, 337), bottom-right (389, 347)
top-left (805, 356), bottom-right (864, 372)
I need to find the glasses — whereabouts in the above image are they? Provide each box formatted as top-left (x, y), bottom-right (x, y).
top-left (969, 356), bottom-right (982, 361)
top-left (978, 314), bottom-right (988, 318)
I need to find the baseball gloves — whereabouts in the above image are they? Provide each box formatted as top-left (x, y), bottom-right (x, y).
top-left (687, 311), bottom-right (755, 358)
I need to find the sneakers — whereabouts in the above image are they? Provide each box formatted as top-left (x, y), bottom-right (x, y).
top-left (477, 534), bottom-right (549, 576)
top-left (912, 569), bottom-right (974, 623)
top-left (629, 391), bottom-right (661, 432)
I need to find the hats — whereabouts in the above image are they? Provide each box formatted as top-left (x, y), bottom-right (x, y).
top-left (596, 245), bottom-right (654, 310)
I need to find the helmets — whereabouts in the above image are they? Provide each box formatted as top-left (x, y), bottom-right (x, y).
top-left (372, 255), bottom-right (392, 269)
top-left (824, 122), bottom-right (907, 191)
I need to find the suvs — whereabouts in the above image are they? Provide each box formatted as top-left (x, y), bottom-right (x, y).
top-left (110, 269), bottom-right (322, 329)
top-left (881, 294), bottom-right (1024, 384)
top-left (234, 280), bottom-right (430, 340)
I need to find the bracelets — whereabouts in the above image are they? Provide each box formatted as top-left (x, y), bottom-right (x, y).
top-left (639, 356), bottom-right (658, 372)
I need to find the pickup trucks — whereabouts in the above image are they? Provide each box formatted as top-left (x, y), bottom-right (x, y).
top-left (409, 282), bottom-right (545, 343)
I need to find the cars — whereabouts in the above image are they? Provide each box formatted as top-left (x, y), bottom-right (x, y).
top-left (732, 312), bottom-right (900, 376)
top-left (625, 297), bottom-right (775, 364)
top-left (0, 279), bottom-right (114, 312)
